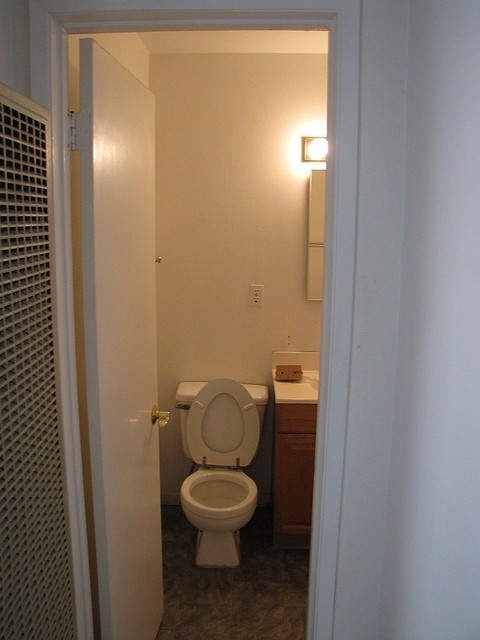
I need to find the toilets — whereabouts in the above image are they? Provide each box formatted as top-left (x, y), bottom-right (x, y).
top-left (175, 378), bottom-right (270, 568)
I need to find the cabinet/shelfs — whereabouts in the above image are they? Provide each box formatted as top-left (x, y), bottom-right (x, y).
top-left (273, 404), bottom-right (320, 555)
